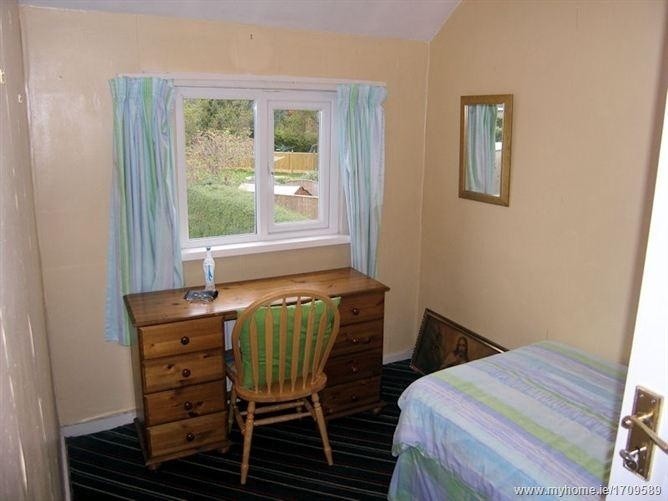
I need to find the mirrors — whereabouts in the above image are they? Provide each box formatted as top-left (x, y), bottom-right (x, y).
top-left (458, 94), bottom-right (513, 207)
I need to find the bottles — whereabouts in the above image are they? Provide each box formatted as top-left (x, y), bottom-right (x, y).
top-left (202, 246), bottom-right (215, 291)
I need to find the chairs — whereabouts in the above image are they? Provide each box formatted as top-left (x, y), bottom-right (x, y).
top-left (224, 289), bottom-right (340, 485)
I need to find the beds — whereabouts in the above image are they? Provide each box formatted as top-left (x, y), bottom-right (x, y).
top-left (387, 340), bottom-right (629, 501)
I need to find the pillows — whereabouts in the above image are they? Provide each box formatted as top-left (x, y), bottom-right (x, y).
top-left (236, 296), bottom-right (342, 391)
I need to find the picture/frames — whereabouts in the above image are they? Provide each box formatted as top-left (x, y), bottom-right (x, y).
top-left (409, 309), bottom-right (509, 375)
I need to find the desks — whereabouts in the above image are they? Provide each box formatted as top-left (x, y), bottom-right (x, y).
top-left (123, 266), bottom-right (390, 470)
